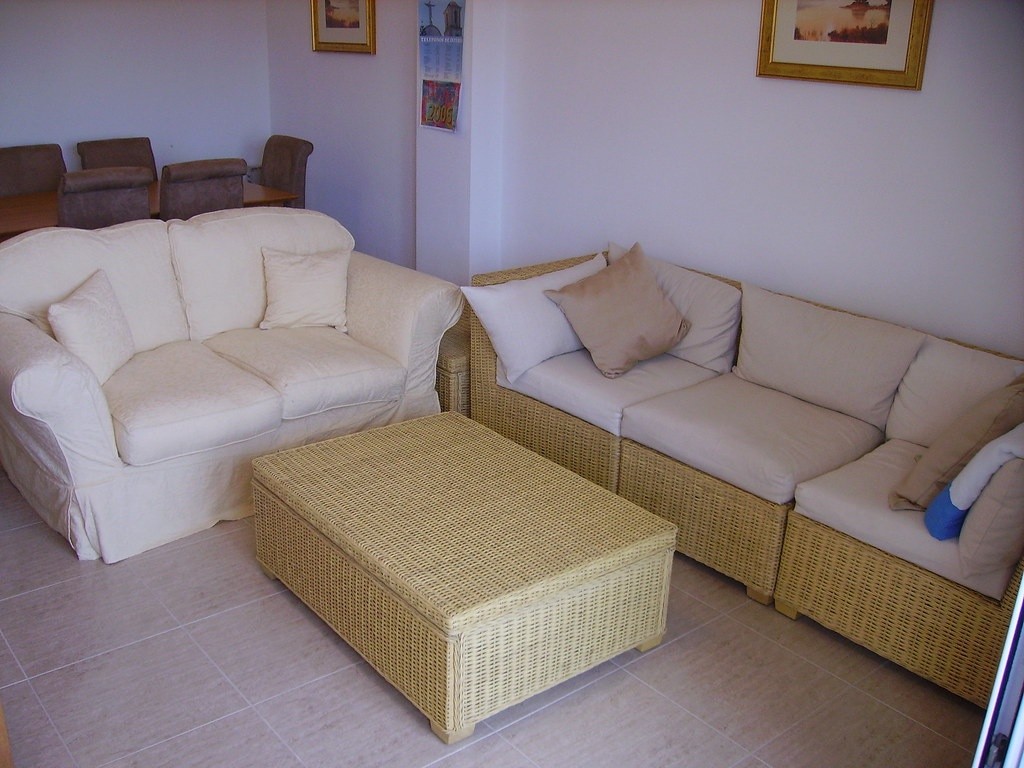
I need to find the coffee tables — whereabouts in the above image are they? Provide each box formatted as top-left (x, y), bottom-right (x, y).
top-left (251, 410), bottom-right (680, 744)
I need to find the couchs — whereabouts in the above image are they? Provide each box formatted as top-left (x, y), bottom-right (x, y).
top-left (470, 239), bottom-right (1024, 714)
top-left (0, 206), bottom-right (466, 567)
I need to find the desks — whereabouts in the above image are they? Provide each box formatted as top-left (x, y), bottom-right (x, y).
top-left (0, 179), bottom-right (298, 241)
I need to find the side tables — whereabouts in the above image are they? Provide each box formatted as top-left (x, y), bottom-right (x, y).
top-left (431, 302), bottom-right (471, 418)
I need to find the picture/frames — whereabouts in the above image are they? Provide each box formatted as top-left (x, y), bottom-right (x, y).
top-left (756, 0), bottom-right (935, 91)
top-left (309, 0), bottom-right (376, 56)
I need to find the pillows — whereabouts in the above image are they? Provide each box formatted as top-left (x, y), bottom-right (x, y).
top-left (735, 282), bottom-right (929, 431)
top-left (888, 372), bottom-right (1021, 512)
top-left (545, 240), bottom-right (693, 379)
top-left (605, 241), bottom-right (743, 376)
top-left (47, 269), bottom-right (136, 386)
top-left (882, 334), bottom-right (1024, 450)
top-left (459, 253), bottom-right (607, 384)
top-left (259, 244), bottom-right (351, 335)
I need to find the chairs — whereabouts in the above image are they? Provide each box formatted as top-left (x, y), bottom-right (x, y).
top-left (57, 168), bottom-right (153, 227)
top-left (1, 143), bottom-right (68, 196)
top-left (249, 135), bottom-right (314, 210)
top-left (159, 158), bottom-right (248, 222)
top-left (77, 137), bottom-right (158, 181)
